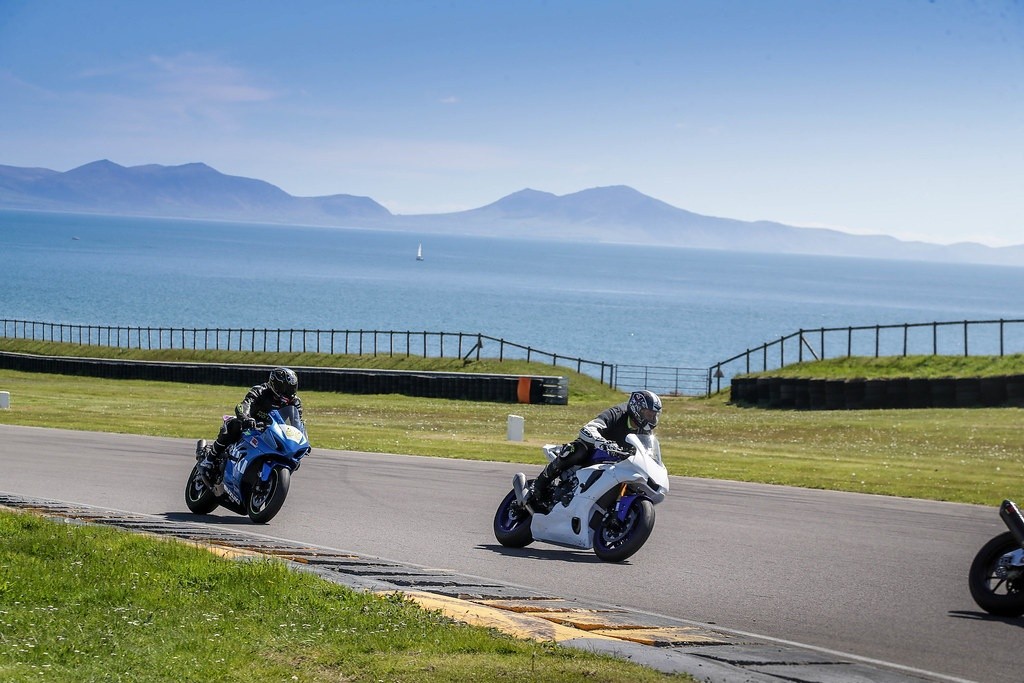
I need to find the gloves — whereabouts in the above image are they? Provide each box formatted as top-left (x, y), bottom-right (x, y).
top-left (599, 440), bottom-right (620, 457)
top-left (242, 417), bottom-right (257, 429)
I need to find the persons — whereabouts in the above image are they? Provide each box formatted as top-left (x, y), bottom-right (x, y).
top-left (199, 367), bottom-right (309, 473)
top-left (530, 390), bottom-right (663, 512)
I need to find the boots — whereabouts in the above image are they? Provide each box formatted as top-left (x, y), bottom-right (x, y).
top-left (200, 438), bottom-right (226, 470)
top-left (531, 465), bottom-right (553, 499)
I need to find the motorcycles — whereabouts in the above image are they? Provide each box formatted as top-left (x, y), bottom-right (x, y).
top-left (492, 433), bottom-right (670, 562)
top-left (968, 499), bottom-right (1024, 619)
top-left (185, 405), bottom-right (312, 524)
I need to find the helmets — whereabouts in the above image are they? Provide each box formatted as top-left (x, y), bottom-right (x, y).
top-left (269, 368), bottom-right (298, 398)
top-left (627, 390), bottom-right (662, 432)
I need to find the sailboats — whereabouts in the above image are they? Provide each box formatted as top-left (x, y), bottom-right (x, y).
top-left (416, 242), bottom-right (424, 260)
